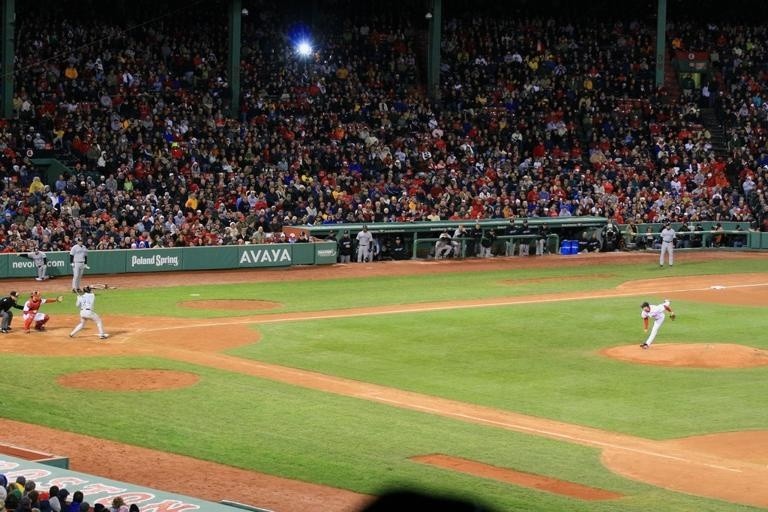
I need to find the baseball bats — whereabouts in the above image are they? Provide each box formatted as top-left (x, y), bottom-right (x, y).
top-left (73, 287), bottom-right (82, 298)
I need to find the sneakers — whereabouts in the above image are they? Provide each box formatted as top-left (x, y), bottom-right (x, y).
top-left (69, 333), bottom-right (112, 337)
top-left (640, 343), bottom-right (648, 350)
top-left (2, 326), bottom-right (13, 334)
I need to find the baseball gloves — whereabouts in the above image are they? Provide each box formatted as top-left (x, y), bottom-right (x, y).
top-left (57, 296), bottom-right (63, 302)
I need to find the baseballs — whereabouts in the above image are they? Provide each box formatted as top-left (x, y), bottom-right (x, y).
top-left (189, 292), bottom-right (199, 296)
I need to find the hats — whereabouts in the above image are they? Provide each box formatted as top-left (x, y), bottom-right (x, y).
top-left (78, 239), bottom-right (82, 242)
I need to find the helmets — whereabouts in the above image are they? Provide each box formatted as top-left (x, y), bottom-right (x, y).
top-left (509, 218), bottom-right (548, 226)
top-left (11, 291), bottom-right (19, 296)
top-left (34, 247), bottom-right (38, 253)
top-left (442, 222), bottom-right (496, 234)
top-left (363, 226), bottom-right (368, 229)
top-left (84, 286), bottom-right (90, 292)
top-left (31, 291), bottom-right (40, 298)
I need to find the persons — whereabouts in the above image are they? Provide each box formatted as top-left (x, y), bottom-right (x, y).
top-left (69, 237), bottom-right (90, 293)
top-left (0, 0), bottom-right (768, 267)
top-left (22, 288), bottom-right (64, 333)
top-left (15, 246), bottom-right (54, 281)
top-left (0, 290), bottom-right (24, 333)
top-left (0, 474), bottom-right (140, 512)
top-left (69, 285), bottom-right (110, 339)
top-left (640, 299), bottom-right (675, 349)
